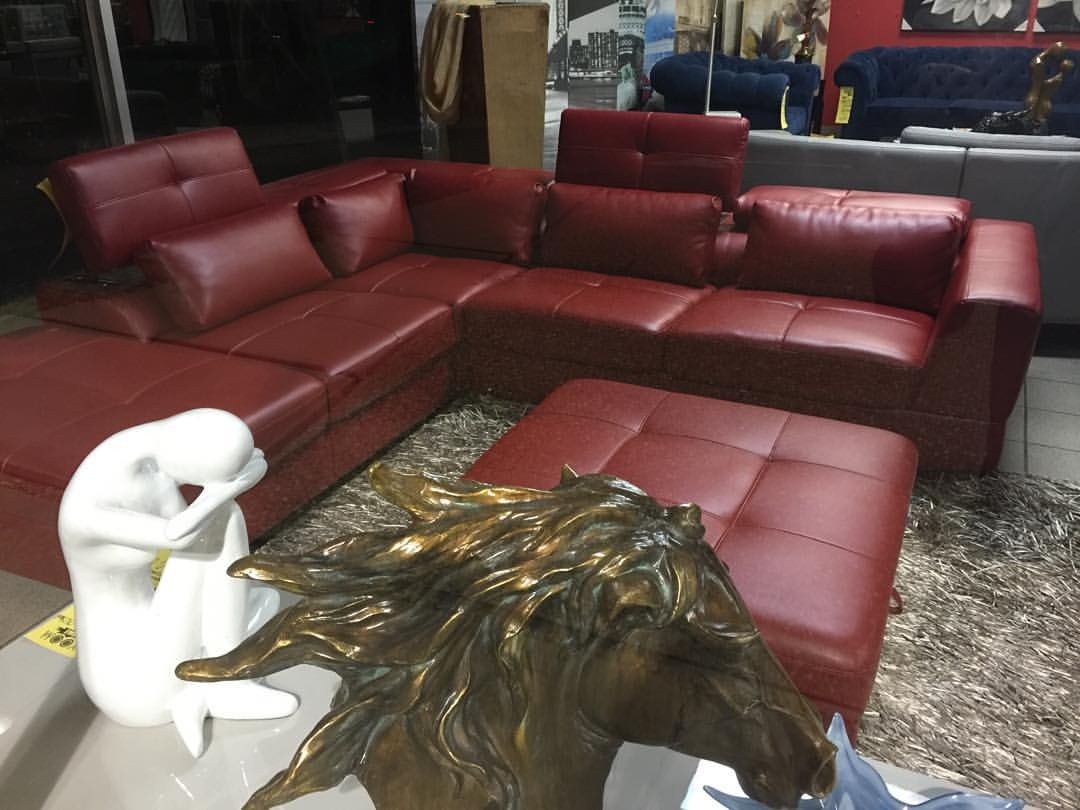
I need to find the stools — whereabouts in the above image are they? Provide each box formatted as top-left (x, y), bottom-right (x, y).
top-left (483, 381), bottom-right (918, 747)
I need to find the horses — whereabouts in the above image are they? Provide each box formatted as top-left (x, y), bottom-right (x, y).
top-left (173, 462), bottom-right (839, 810)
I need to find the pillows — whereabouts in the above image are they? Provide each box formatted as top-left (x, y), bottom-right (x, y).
top-left (543, 183), bottom-right (722, 288)
top-left (134, 201), bottom-right (334, 331)
top-left (302, 171), bottom-right (414, 278)
top-left (408, 168), bottom-right (545, 271)
top-left (737, 201), bottom-right (960, 315)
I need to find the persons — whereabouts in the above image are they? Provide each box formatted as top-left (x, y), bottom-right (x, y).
top-left (56, 408), bottom-right (299, 757)
top-left (1023, 41), bottom-right (1075, 122)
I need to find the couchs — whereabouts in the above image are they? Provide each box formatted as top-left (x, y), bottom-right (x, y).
top-left (2, 48), bottom-right (1080, 589)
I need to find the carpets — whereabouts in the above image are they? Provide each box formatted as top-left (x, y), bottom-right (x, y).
top-left (246, 396), bottom-right (1079, 810)
